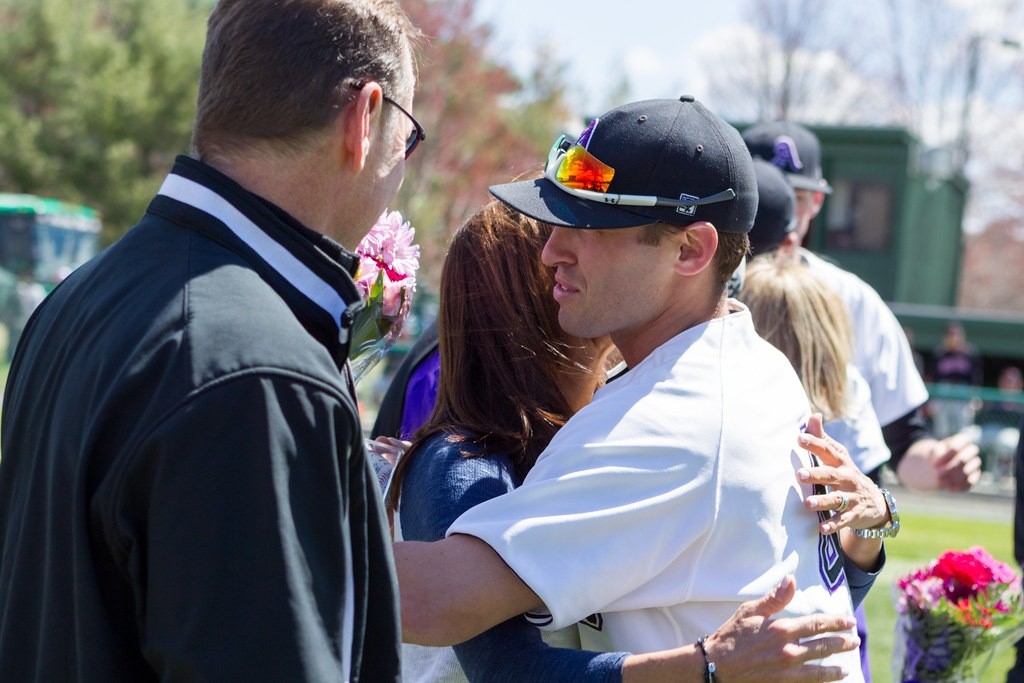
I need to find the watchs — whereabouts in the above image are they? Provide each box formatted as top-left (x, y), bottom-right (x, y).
top-left (850, 484), bottom-right (900, 539)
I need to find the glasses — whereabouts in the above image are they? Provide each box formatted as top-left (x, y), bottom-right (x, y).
top-left (349, 79), bottom-right (425, 160)
top-left (543, 135), bottom-right (736, 207)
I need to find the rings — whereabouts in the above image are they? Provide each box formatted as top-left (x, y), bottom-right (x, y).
top-left (834, 491), bottom-right (848, 511)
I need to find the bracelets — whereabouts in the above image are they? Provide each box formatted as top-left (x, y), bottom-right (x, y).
top-left (696, 635), bottom-right (717, 683)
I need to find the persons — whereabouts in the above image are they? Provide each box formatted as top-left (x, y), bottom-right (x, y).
top-left (740, 125), bottom-right (981, 494)
top-left (974, 367), bottom-right (1024, 484)
top-left (400, 204), bottom-right (899, 683)
top-left (730, 157), bottom-right (892, 683)
top-left (371, 317), bottom-right (441, 439)
top-left (0, 0), bottom-right (427, 683)
top-left (925, 324), bottom-right (981, 435)
top-left (372, 94), bottom-right (865, 683)
top-left (737, 264), bottom-right (846, 420)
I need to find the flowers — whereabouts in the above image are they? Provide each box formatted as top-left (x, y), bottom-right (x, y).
top-left (347, 206), bottom-right (420, 387)
top-left (889, 546), bottom-right (1024, 683)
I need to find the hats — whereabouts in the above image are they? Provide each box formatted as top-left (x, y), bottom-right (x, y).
top-left (489, 95), bottom-right (759, 232)
top-left (748, 155), bottom-right (796, 241)
top-left (743, 119), bottom-right (833, 194)
